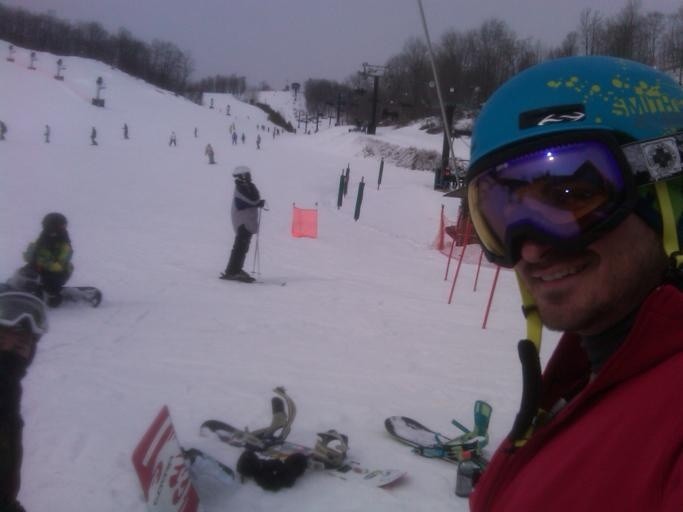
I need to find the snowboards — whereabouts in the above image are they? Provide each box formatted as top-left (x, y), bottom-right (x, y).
top-left (199, 384), bottom-right (408, 488)
top-left (132, 406), bottom-right (236, 511)
top-left (0, 282), bottom-right (101, 307)
top-left (384, 399), bottom-right (493, 476)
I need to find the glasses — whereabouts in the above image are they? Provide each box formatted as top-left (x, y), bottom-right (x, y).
top-left (468, 142), bottom-right (645, 257)
top-left (0, 291), bottom-right (47, 335)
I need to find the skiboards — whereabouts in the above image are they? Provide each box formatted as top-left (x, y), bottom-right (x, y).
top-left (218, 272), bottom-right (286, 286)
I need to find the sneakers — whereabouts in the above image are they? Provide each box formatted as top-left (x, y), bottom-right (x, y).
top-left (225, 269), bottom-right (256, 281)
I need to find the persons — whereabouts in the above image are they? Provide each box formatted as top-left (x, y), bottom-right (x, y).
top-left (232, 132), bottom-right (237, 144)
top-left (0, 292), bottom-right (49, 512)
top-left (467, 53), bottom-right (683, 512)
top-left (225, 165), bottom-right (265, 275)
top-left (169, 131), bottom-right (176, 146)
top-left (23, 213), bottom-right (74, 308)
top-left (256, 134), bottom-right (261, 149)
top-left (205, 143), bottom-right (215, 164)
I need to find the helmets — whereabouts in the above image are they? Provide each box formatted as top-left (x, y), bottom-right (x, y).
top-left (43, 212), bottom-right (66, 230)
top-left (231, 165), bottom-right (249, 177)
top-left (471, 56), bottom-right (683, 169)
